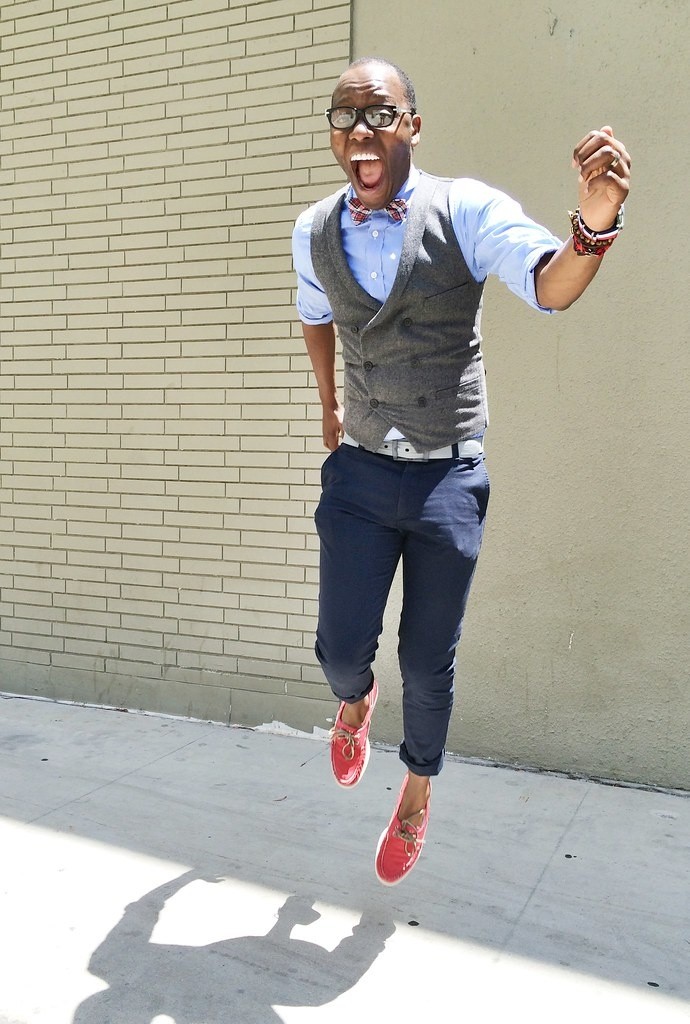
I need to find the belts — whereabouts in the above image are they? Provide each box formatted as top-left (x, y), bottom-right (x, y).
top-left (341, 426), bottom-right (485, 462)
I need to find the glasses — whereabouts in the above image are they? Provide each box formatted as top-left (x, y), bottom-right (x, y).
top-left (325, 104), bottom-right (412, 130)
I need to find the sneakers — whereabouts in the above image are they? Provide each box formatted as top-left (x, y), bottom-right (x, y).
top-left (375, 771), bottom-right (432, 887)
top-left (329, 679), bottom-right (379, 789)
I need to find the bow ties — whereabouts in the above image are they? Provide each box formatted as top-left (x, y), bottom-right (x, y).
top-left (348, 195), bottom-right (407, 226)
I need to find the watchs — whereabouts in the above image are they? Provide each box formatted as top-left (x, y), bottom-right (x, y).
top-left (579, 204), bottom-right (627, 241)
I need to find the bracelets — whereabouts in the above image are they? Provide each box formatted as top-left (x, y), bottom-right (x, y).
top-left (569, 208), bottom-right (615, 258)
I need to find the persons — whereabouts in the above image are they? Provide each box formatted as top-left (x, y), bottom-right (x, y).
top-left (292, 59), bottom-right (631, 885)
